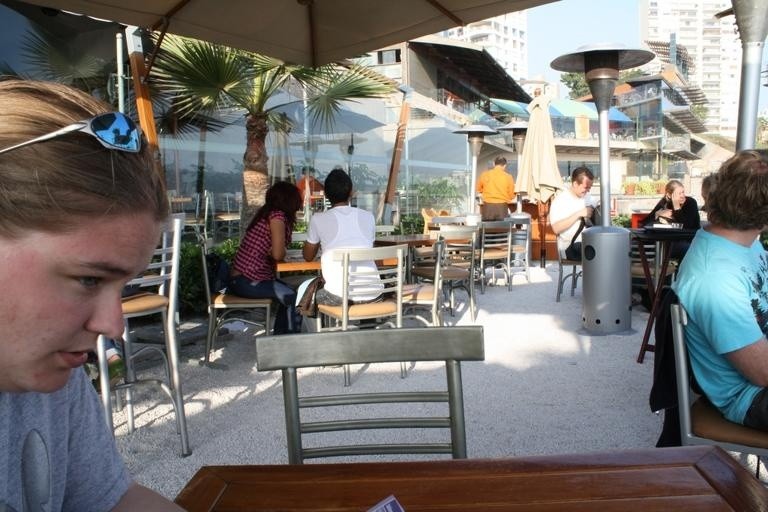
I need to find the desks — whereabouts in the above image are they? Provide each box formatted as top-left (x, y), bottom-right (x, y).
top-left (630, 221), bottom-right (710, 363)
top-left (171, 444), bottom-right (766, 511)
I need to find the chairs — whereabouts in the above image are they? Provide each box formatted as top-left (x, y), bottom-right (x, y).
top-left (554, 234), bottom-right (583, 303)
top-left (96, 208), bottom-right (533, 465)
top-left (630, 234), bottom-right (678, 312)
top-left (670, 305), bottom-right (768, 470)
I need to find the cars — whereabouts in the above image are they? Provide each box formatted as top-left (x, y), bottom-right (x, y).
top-left (668, 162), bottom-right (689, 178)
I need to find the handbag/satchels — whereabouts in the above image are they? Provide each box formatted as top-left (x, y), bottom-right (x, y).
top-left (565, 205), bottom-right (598, 262)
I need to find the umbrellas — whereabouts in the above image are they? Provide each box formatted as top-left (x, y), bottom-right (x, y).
top-left (514, 89), bottom-right (564, 267)
top-left (23, 0), bottom-right (563, 73)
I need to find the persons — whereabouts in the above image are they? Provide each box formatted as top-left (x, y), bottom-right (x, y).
top-left (294, 166), bottom-right (324, 210)
top-left (670, 149), bottom-right (767, 432)
top-left (0, 78), bottom-right (188, 512)
top-left (549, 166), bottom-right (599, 261)
top-left (475, 155), bottom-right (516, 268)
top-left (301, 168), bottom-right (385, 332)
top-left (640, 180), bottom-right (700, 258)
top-left (226, 181), bottom-right (304, 335)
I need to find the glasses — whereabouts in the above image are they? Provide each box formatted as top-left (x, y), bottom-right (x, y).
top-left (0, 108), bottom-right (149, 155)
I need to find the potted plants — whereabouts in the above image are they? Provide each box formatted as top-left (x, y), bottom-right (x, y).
top-left (646, 179), bottom-right (666, 193)
top-left (619, 180), bottom-right (642, 195)
